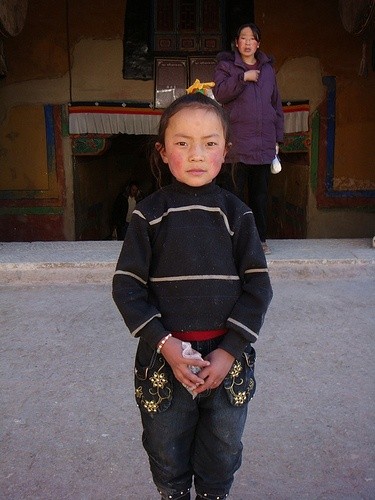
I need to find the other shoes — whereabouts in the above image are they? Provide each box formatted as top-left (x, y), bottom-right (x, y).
top-left (261, 242), bottom-right (271, 254)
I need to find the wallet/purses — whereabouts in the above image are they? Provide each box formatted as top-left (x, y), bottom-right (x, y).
top-left (270, 155), bottom-right (281, 174)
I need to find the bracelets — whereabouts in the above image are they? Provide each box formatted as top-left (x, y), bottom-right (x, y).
top-left (156, 333), bottom-right (172, 354)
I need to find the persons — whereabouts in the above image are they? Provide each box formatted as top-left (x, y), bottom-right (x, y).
top-left (113, 185), bottom-right (143, 241)
top-left (212, 23), bottom-right (284, 255)
top-left (112, 79), bottom-right (273, 500)
